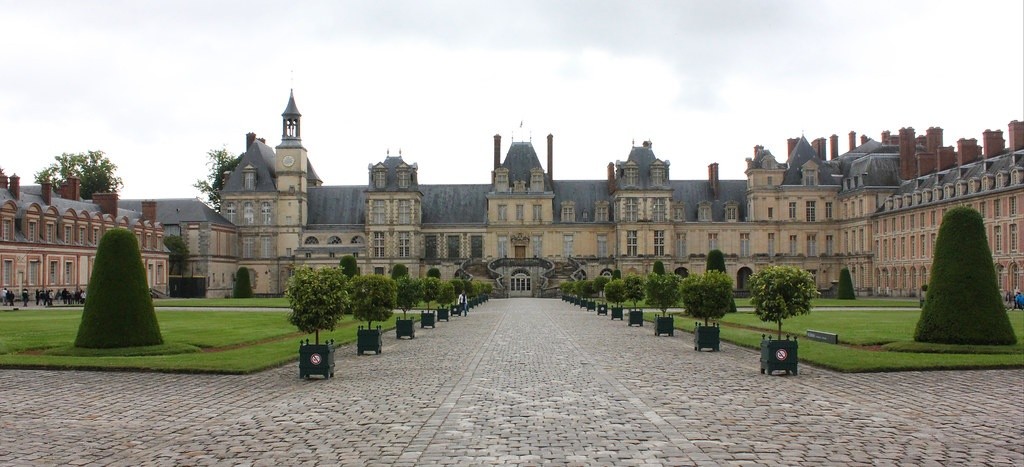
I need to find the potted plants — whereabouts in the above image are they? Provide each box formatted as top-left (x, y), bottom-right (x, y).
top-left (747, 266), bottom-right (815, 375)
top-left (285, 265), bottom-right (351, 379)
top-left (418, 277), bottom-right (492, 328)
top-left (560, 275), bottom-right (646, 326)
top-left (347, 273), bottom-right (397, 354)
top-left (681, 269), bottom-right (734, 352)
top-left (644, 272), bottom-right (686, 335)
top-left (396, 275), bottom-right (425, 339)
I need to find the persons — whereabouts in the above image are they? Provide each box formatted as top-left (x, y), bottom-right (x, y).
top-left (0, 287), bottom-right (86, 307)
top-left (458, 289), bottom-right (468, 317)
